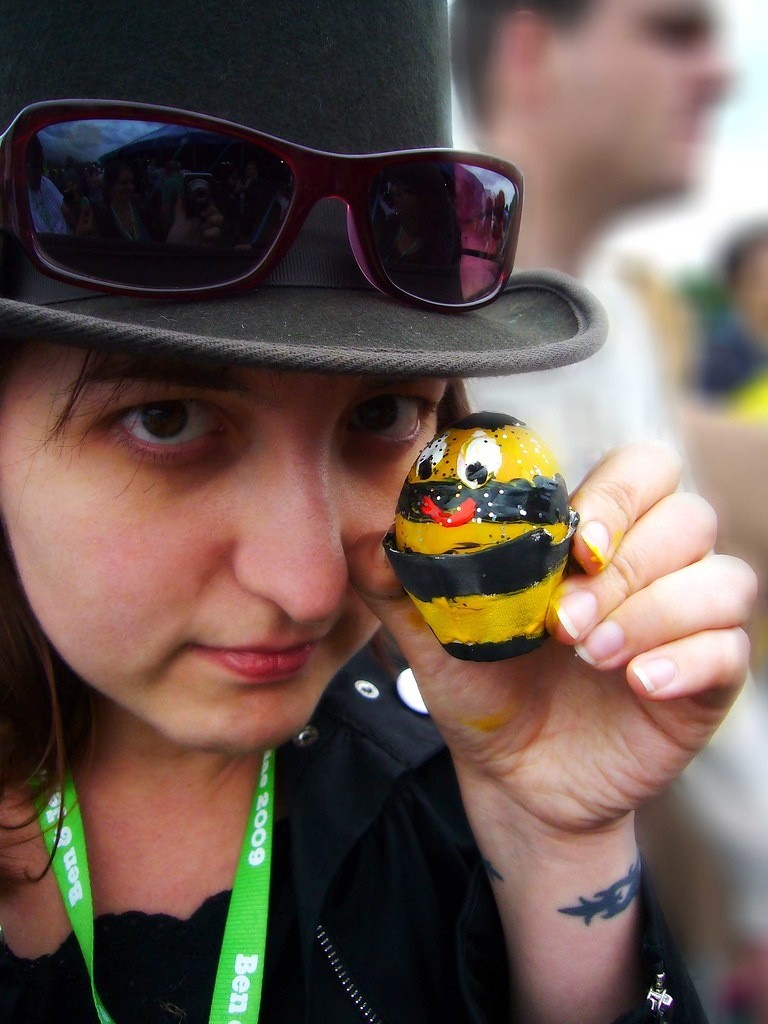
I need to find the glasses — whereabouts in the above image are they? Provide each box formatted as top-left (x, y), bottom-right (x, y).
top-left (2, 99), bottom-right (524, 311)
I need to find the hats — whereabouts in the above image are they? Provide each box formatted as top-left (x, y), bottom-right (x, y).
top-left (0, 0), bottom-right (609, 374)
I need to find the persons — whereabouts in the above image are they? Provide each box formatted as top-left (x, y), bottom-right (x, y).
top-left (448, 1), bottom-right (768, 1023)
top-left (0, 3), bottom-right (759, 1023)
